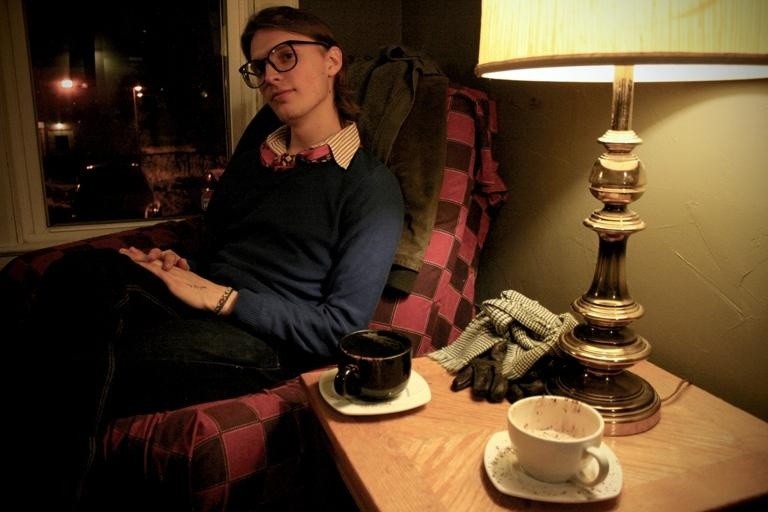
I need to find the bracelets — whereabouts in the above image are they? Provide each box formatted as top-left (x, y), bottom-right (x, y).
top-left (212, 286), bottom-right (234, 315)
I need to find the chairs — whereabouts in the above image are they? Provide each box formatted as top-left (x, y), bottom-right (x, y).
top-left (0, 85), bottom-right (511, 512)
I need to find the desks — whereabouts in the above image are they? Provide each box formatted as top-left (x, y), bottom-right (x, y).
top-left (300, 359), bottom-right (768, 512)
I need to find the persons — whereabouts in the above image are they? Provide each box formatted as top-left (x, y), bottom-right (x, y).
top-left (0, 5), bottom-right (406, 512)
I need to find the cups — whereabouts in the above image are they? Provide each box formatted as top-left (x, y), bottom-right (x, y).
top-left (332, 330), bottom-right (412, 401)
top-left (508, 395), bottom-right (610, 488)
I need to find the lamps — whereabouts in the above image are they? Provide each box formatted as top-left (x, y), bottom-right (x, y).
top-left (473, 0), bottom-right (768, 436)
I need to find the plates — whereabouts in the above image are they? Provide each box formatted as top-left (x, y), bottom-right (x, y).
top-left (318, 367), bottom-right (432, 419)
top-left (484, 428), bottom-right (624, 503)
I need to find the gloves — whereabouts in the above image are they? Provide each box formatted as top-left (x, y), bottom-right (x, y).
top-left (450, 350), bottom-right (548, 405)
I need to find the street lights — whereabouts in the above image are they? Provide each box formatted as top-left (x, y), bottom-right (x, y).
top-left (133, 84), bottom-right (143, 132)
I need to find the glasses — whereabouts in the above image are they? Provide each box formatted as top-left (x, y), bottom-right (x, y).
top-left (239, 40), bottom-right (328, 88)
